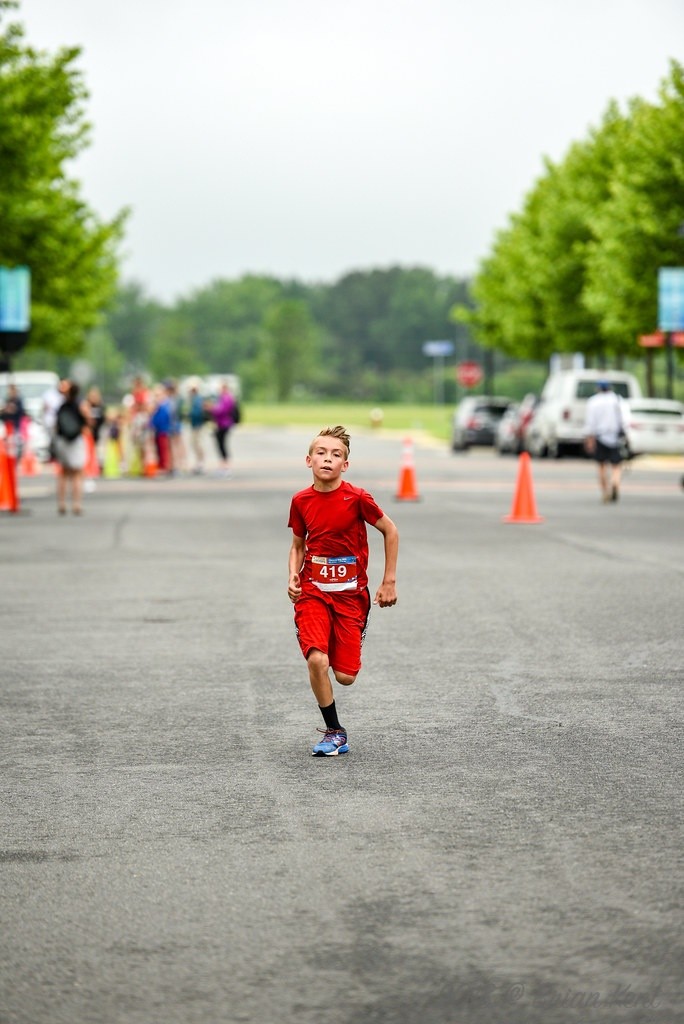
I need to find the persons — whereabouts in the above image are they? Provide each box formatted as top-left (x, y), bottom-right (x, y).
top-left (584, 380), bottom-right (628, 505)
top-left (187, 386), bottom-right (208, 477)
top-left (0, 378), bottom-right (185, 476)
top-left (47, 383), bottom-right (84, 519)
top-left (202, 383), bottom-right (238, 479)
top-left (285, 425), bottom-right (400, 759)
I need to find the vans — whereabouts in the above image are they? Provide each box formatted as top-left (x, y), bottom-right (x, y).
top-left (0, 372), bottom-right (66, 436)
top-left (528, 368), bottom-right (642, 461)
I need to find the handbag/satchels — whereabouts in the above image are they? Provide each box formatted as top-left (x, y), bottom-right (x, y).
top-left (51, 434), bottom-right (86, 470)
top-left (618, 430), bottom-right (631, 458)
top-left (233, 407), bottom-right (240, 423)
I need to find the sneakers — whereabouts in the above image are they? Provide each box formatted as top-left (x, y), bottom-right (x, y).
top-left (360, 613), bottom-right (371, 647)
top-left (312, 728), bottom-right (349, 756)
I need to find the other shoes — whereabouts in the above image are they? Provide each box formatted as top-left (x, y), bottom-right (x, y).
top-left (611, 482), bottom-right (619, 500)
top-left (59, 509), bottom-right (66, 513)
top-left (74, 509), bottom-right (85, 515)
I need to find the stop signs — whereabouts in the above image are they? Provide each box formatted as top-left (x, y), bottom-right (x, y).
top-left (455, 362), bottom-right (480, 387)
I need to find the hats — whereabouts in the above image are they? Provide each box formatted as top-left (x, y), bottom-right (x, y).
top-left (600, 381), bottom-right (609, 388)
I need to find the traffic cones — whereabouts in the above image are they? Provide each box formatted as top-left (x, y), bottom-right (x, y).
top-left (392, 440), bottom-right (422, 502)
top-left (502, 454), bottom-right (542, 525)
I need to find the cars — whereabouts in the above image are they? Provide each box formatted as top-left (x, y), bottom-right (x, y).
top-left (620, 397), bottom-right (684, 459)
top-left (452, 396), bottom-right (542, 457)
